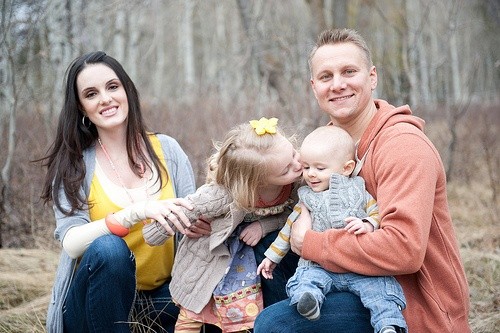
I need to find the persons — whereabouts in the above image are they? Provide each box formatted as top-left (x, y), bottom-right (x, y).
top-left (256, 125), bottom-right (408, 333)
top-left (254, 28), bottom-right (472, 333)
top-left (31, 50), bottom-right (210, 333)
top-left (142, 117), bottom-right (305, 333)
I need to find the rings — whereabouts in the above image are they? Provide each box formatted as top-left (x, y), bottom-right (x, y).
top-left (165, 213), bottom-right (171, 218)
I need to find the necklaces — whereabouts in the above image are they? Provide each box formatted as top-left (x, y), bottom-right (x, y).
top-left (96, 138), bottom-right (147, 204)
